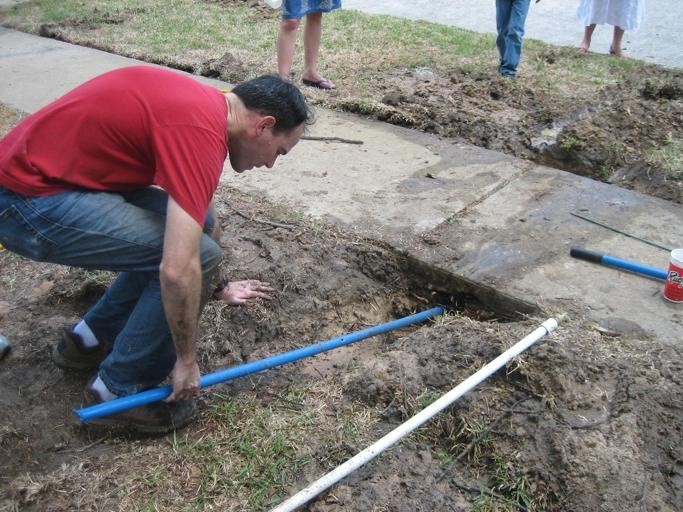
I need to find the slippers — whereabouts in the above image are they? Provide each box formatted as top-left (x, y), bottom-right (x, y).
top-left (303, 77), bottom-right (334, 89)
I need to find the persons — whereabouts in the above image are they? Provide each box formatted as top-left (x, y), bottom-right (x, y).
top-left (576, 0), bottom-right (645, 61)
top-left (274, 0), bottom-right (343, 91)
top-left (494, 0), bottom-right (541, 80)
top-left (0, 64), bottom-right (318, 433)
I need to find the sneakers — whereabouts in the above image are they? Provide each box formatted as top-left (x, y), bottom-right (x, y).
top-left (54, 322), bottom-right (106, 373)
top-left (80, 374), bottom-right (198, 434)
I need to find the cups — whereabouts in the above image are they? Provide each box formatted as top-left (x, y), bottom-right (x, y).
top-left (663, 249), bottom-right (683, 303)
